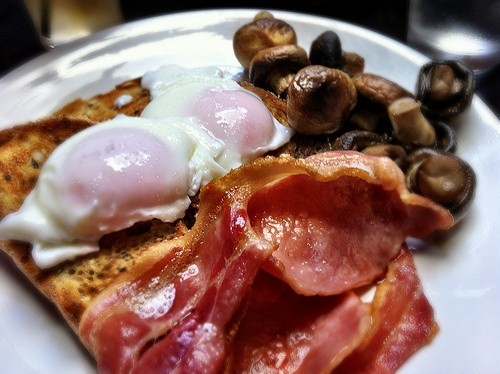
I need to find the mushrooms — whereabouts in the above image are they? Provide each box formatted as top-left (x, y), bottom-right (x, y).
top-left (232, 11), bottom-right (478, 225)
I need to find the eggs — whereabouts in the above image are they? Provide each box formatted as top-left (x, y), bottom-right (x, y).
top-left (1, 67), bottom-right (294, 273)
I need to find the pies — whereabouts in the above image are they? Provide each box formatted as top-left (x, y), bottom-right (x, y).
top-left (0, 77), bottom-right (296, 340)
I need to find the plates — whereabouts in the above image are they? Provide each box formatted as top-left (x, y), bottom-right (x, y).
top-left (0, 8), bottom-right (499, 374)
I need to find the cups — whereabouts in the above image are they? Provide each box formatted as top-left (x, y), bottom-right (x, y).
top-left (24, 0), bottom-right (123, 51)
top-left (408, 0), bottom-right (499, 75)
